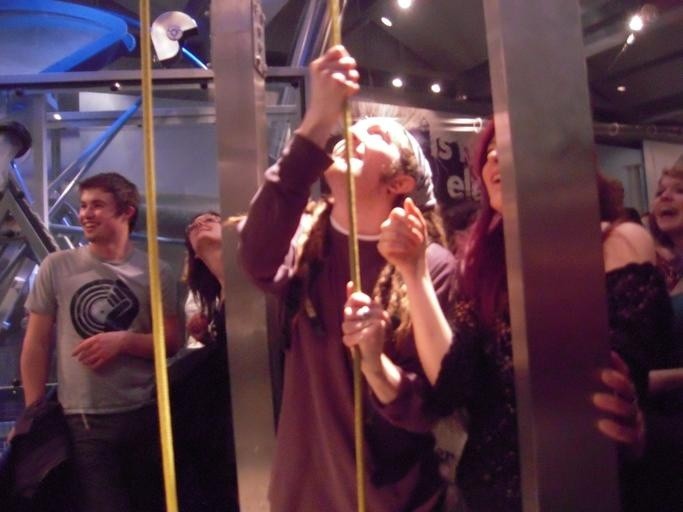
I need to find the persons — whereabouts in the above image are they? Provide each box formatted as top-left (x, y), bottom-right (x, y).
top-left (236, 46), bottom-right (682, 511)
top-left (20, 173), bottom-right (184, 511)
top-left (182, 211), bottom-right (235, 511)
